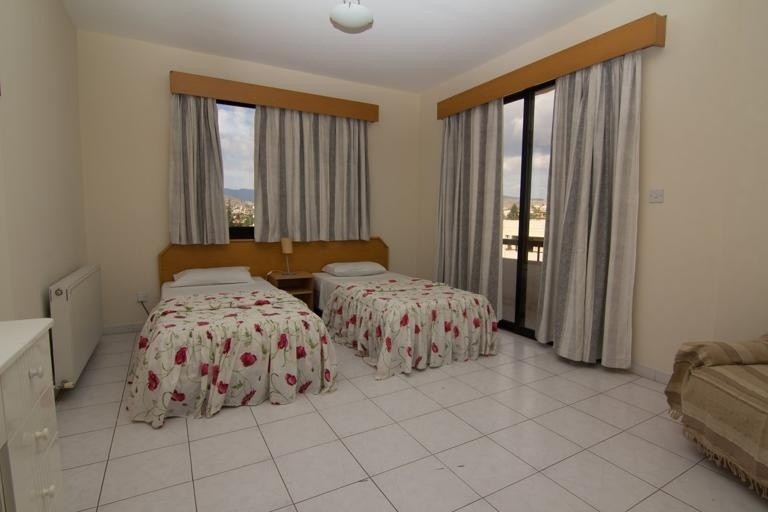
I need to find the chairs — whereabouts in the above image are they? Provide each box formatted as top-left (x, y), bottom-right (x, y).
top-left (664, 333), bottom-right (768, 501)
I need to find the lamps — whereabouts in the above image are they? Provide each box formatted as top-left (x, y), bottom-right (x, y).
top-left (280, 237), bottom-right (298, 277)
top-left (330, 0), bottom-right (375, 30)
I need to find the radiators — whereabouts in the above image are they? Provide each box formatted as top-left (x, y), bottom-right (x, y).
top-left (47, 262), bottom-right (106, 390)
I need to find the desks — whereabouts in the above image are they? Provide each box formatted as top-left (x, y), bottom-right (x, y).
top-left (0, 318), bottom-right (67, 511)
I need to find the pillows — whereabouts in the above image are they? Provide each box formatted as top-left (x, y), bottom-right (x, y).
top-left (167, 266), bottom-right (255, 287)
top-left (321, 260), bottom-right (388, 278)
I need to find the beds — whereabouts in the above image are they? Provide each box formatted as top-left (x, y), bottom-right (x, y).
top-left (124, 271), bottom-right (339, 430)
top-left (310, 271), bottom-right (499, 381)
